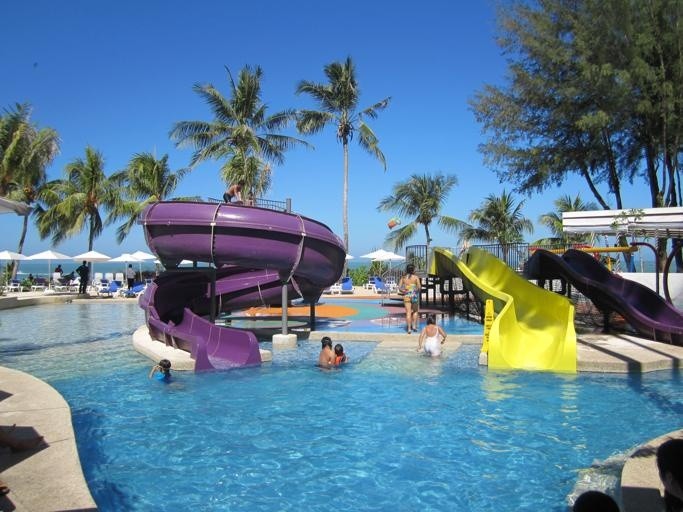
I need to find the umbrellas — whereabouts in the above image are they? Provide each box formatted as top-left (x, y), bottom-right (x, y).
top-left (72, 251), bottom-right (111, 279)
top-left (372, 251), bottom-right (406, 274)
top-left (358, 247), bottom-right (389, 276)
top-left (130, 250), bottom-right (156, 282)
top-left (108, 253), bottom-right (144, 282)
top-left (0, 250), bottom-right (32, 281)
top-left (28, 250), bottom-right (71, 281)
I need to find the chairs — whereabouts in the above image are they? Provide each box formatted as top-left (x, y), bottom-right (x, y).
top-left (4, 271), bottom-right (81, 293)
top-left (362, 275), bottom-right (398, 296)
top-left (323, 274), bottom-right (355, 296)
top-left (88, 272), bottom-right (153, 299)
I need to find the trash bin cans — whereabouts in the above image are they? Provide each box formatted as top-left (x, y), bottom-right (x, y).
top-left (374, 277), bottom-right (383, 288)
top-left (342, 277), bottom-right (351, 290)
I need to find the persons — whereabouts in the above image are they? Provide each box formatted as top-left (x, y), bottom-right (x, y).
top-left (126, 263), bottom-right (135, 295)
top-left (397, 263), bottom-right (421, 334)
top-left (318, 336), bottom-right (335, 368)
top-left (222, 180), bottom-right (244, 203)
top-left (54, 264), bottom-right (63, 275)
top-left (149, 360), bottom-right (185, 390)
top-left (27, 273), bottom-right (36, 282)
top-left (245, 192), bottom-right (253, 207)
top-left (334, 344), bottom-right (349, 367)
top-left (74, 259), bottom-right (88, 293)
top-left (417, 315), bottom-right (445, 358)
